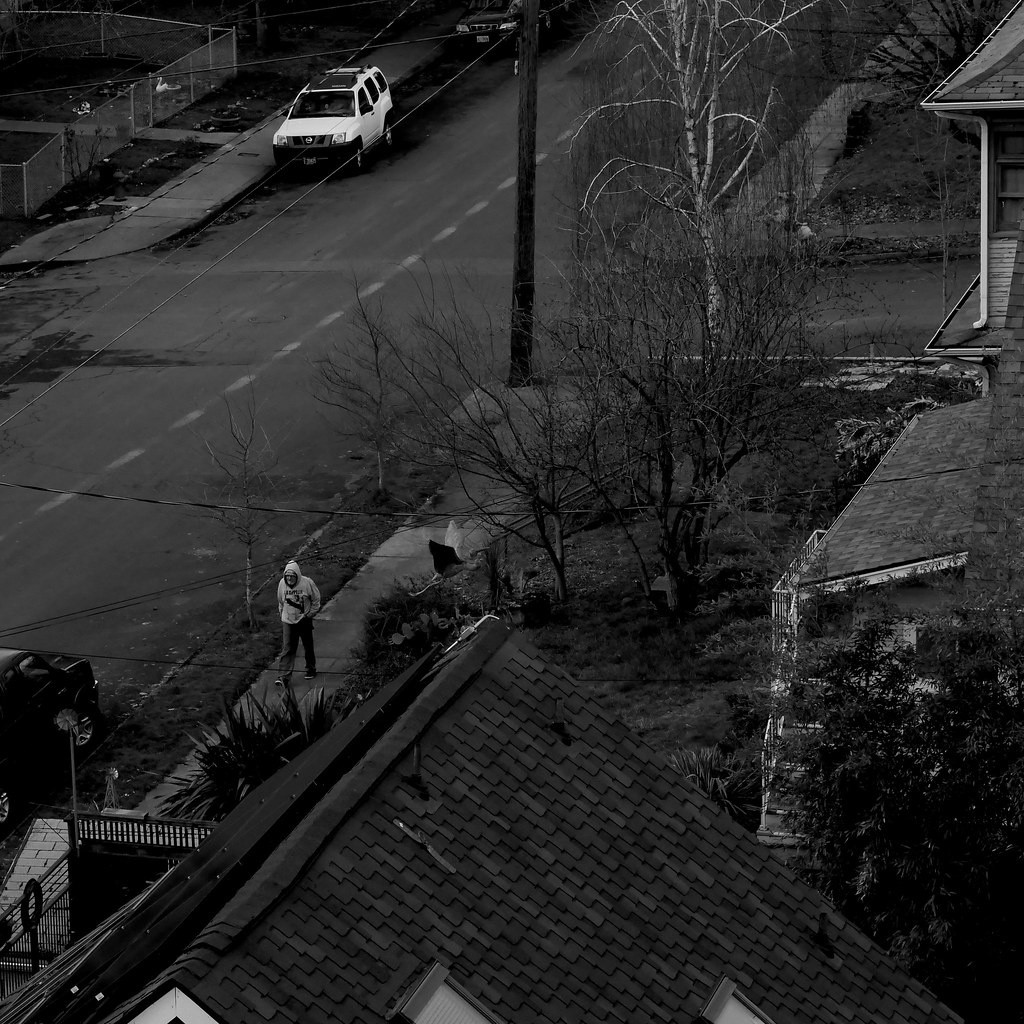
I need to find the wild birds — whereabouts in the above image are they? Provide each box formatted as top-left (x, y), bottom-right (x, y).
top-left (405, 520), bottom-right (490, 597)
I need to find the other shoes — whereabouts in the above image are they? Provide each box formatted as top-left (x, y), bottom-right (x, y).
top-left (275, 677), bottom-right (288, 688)
top-left (304, 671), bottom-right (317, 680)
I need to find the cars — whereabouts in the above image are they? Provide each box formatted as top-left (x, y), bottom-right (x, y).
top-left (0, 649), bottom-right (100, 828)
top-left (456, 0), bottom-right (570, 51)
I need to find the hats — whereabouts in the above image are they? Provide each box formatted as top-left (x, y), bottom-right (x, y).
top-left (284, 570), bottom-right (296, 577)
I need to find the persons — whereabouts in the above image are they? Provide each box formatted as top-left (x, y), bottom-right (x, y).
top-left (275, 560), bottom-right (321, 686)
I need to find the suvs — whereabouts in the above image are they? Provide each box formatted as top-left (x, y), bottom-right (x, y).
top-left (273, 64), bottom-right (393, 173)
